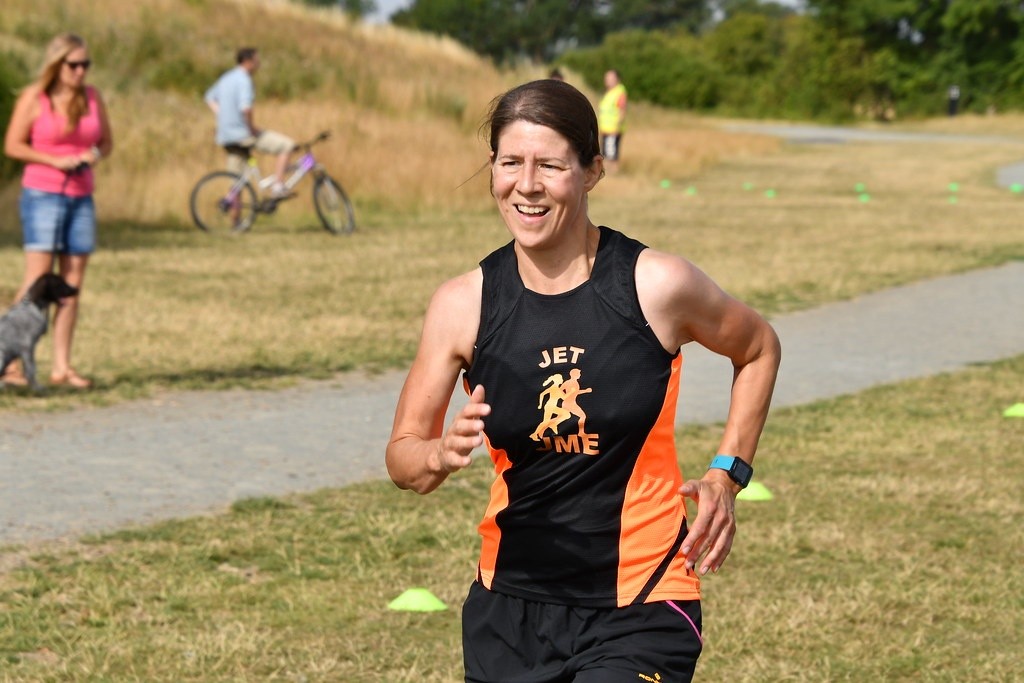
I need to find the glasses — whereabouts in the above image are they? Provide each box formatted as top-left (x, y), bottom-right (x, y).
top-left (64, 58), bottom-right (90, 69)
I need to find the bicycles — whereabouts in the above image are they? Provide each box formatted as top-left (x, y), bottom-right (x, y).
top-left (185, 127), bottom-right (355, 240)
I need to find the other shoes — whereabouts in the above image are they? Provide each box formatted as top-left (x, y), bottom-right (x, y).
top-left (51, 368), bottom-right (92, 387)
top-left (2, 361), bottom-right (27, 385)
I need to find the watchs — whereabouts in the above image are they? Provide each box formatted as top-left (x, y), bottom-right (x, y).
top-left (709, 455), bottom-right (753, 488)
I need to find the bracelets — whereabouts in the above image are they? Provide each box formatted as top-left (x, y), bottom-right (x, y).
top-left (90, 146), bottom-right (102, 159)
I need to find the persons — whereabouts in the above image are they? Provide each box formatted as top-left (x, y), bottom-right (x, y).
top-left (385, 79), bottom-right (781, 683)
top-left (593, 68), bottom-right (628, 174)
top-left (202, 45), bottom-right (292, 232)
top-left (0, 33), bottom-right (114, 389)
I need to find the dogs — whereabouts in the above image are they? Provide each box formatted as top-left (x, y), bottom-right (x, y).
top-left (0, 271), bottom-right (78, 394)
